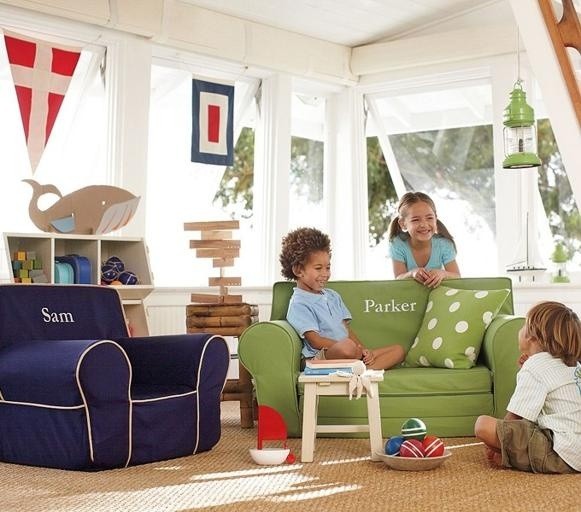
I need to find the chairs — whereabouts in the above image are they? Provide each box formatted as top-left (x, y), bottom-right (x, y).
top-left (0, 285), bottom-right (230, 471)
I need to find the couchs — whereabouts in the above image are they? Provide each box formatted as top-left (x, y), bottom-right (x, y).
top-left (236, 277), bottom-right (530, 440)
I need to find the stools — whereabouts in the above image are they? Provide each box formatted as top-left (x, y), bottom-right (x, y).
top-left (300, 370), bottom-right (385, 462)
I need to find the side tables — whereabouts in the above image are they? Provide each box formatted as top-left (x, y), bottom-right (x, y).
top-left (185, 303), bottom-right (262, 429)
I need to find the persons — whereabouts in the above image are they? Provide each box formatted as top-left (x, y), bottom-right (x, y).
top-left (278, 228), bottom-right (406, 374)
top-left (473, 300), bottom-right (580, 475)
top-left (387, 191), bottom-right (461, 290)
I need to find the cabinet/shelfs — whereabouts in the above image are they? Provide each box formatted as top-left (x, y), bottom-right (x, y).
top-left (4, 232), bottom-right (154, 338)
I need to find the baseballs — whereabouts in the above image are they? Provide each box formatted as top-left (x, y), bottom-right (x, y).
top-left (385, 418), bottom-right (444, 457)
top-left (101, 256), bottom-right (137, 285)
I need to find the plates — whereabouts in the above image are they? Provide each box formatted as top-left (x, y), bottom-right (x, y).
top-left (376, 448), bottom-right (452, 470)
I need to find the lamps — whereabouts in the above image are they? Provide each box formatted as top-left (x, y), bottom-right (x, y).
top-left (502, 25), bottom-right (543, 170)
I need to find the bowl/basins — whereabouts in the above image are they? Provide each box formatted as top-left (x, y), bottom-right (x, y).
top-left (249, 447), bottom-right (289, 465)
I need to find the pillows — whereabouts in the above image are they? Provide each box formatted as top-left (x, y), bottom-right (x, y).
top-left (403, 284), bottom-right (511, 369)
